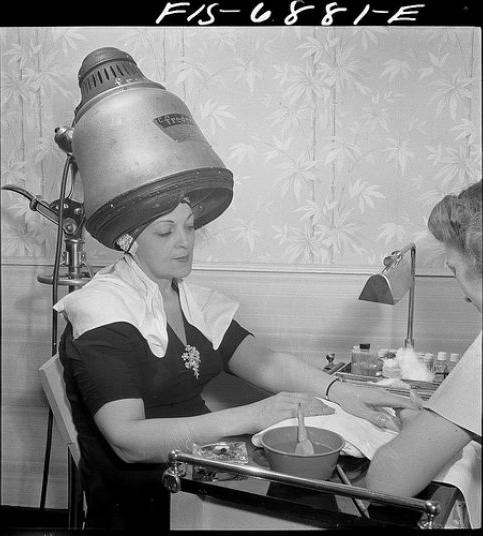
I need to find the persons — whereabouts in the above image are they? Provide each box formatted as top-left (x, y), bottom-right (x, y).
top-left (363, 179), bottom-right (483, 531)
top-left (53, 193), bottom-right (416, 536)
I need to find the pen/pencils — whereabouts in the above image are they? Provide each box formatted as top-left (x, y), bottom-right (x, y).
top-left (336, 463), bottom-right (371, 520)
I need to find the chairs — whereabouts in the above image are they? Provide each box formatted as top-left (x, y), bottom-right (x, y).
top-left (37, 353), bottom-right (79, 465)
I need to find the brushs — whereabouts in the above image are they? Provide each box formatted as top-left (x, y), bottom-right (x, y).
top-left (294, 402), bottom-right (316, 456)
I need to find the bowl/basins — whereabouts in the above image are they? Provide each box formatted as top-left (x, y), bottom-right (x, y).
top-left (261, 425), bottom-right (346, 481)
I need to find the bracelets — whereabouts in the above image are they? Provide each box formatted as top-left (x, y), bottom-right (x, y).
top-left (325, 376), bottom-right (339, 400)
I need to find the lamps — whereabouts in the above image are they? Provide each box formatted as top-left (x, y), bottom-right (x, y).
top-left (357, 242), bottom-right (436, 384)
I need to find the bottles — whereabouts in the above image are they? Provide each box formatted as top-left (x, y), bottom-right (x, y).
top-left (352, 343), bottom-right (461, 382)
